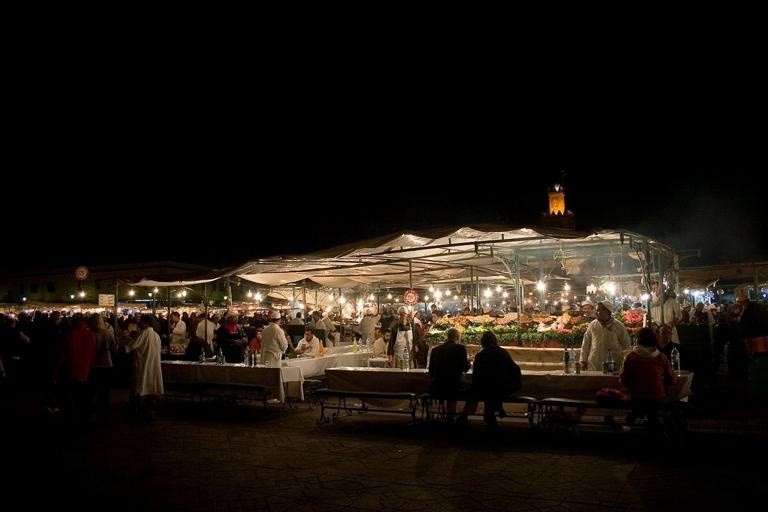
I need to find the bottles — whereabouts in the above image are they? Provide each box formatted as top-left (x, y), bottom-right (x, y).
top-left (319, 339), bottom-right (324, 357)
top-left (399, 346), bottom-right (412, 372)
top-left (198, 345), bottom-right (257, 367)
top-left (671, 346), bottom-right (680, 377)
top-left (562, 346), bottom-right (616, 375)
top-left (351, 336), bottom-right (371, 353)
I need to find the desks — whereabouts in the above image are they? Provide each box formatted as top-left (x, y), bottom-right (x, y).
top-left (462, 344), bottom-right (694, 407)
top-left (279, 349), bottom-right (370, 390)
top-left (161, 360), bottom-right (286, 405)
top-left (324, 366), bottom-right (433, 421)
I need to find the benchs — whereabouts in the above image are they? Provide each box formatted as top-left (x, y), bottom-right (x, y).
top-left (313, 389), bottom-right (423, 428)
top-left (163, 381), bottom-right (270, 410)
top-left (420, 393), bottom-right (690, 439)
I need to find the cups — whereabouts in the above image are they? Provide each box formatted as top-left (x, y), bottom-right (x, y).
top-left (264, 354), bottom-right (301, 367)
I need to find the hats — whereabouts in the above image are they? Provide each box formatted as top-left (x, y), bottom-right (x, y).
top-left (397, 306), bottom-right (409, 314)
top-left (224, 310), bottom-right (241, 320)
top-left (600, 299), bottom-right (614, 313)
top-left (270, 311), bottom-right (282, 320)
